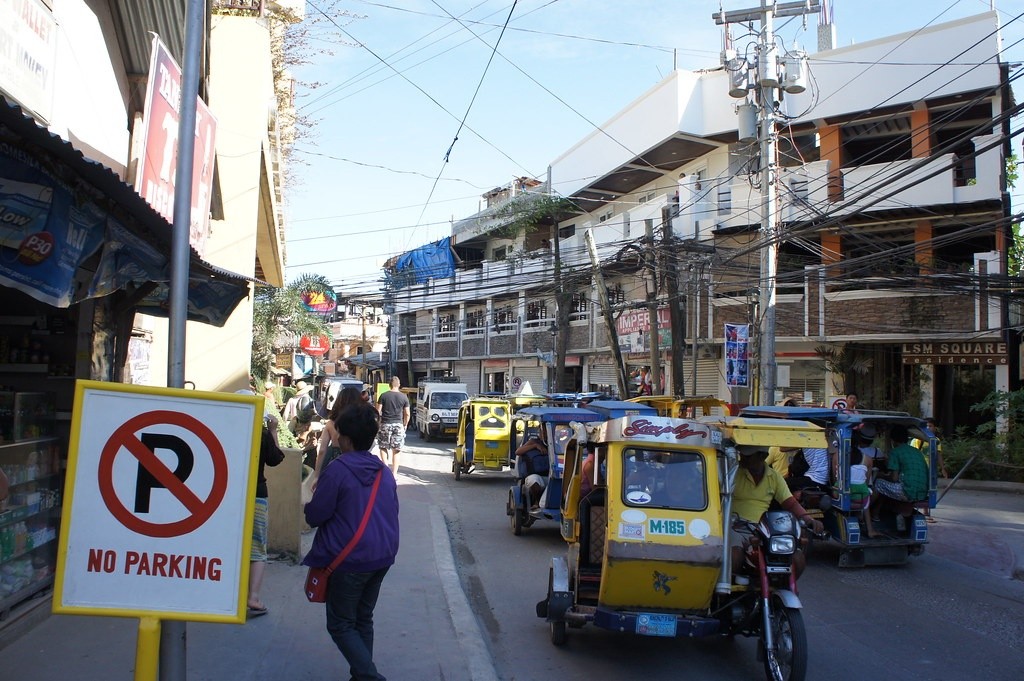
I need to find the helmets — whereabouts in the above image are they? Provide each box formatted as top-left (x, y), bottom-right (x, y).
top-left (297, 397), bottom-right (313, 423)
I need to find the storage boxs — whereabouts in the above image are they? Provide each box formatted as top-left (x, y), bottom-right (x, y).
top-left (0, 461), bottom-right (62, 580)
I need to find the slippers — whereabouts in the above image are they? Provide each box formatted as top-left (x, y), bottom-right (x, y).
top-left (247, 601), bottom-right (269, 614)
top-left (869, 530), bottom-right (885, 539)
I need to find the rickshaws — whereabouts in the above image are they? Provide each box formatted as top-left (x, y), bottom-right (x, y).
top-left (622, 394), bottom-right (733, 419)
top-left (506, 400), bottom-right (659, 537)
top-left (736, 404), bottom-right (942, 570)
top-left (451, 393), bottom-right (562, 481)
top-left (536, 414), bottom-right (828, 681)
top-left (541, 391), bottom-right (622, 409)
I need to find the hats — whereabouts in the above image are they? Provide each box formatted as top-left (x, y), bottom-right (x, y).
top-left (857, 423), bottom-right (877, 439)
top-left (235, 389), bottom-right (270, 420)
top-left (737, 446), bottom-right (769, 455)
top-left (294, 381), bottom-right (314, 395)
top-left (265, 383), bottom-right (275, 389)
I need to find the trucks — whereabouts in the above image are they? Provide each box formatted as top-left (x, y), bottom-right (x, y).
top-left (414, 382), bottom-right (472, 444)
top-left (319, 375), bottom-right (366, 418)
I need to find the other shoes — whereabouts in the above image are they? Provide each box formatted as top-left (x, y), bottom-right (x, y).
top-left (926, 516), bottom-right (937, 523)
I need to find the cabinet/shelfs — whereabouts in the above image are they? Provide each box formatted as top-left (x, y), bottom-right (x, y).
top-left (0, 433), bottom-right (68, 621)
top-left (0, 314), bottom-right (71, 422)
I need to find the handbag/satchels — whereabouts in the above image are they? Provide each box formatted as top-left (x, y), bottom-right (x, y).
top-left (303, 566), bottom-right (329, 603)
top-left (525, 447), bottom-right (548, 476)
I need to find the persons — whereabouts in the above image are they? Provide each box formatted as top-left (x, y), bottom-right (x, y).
top-left (282, 381), bottom-right (314, 422)
top-left (910, 419), bottom-right (947, 522)
top-left (289, 410), bottom-right (317, 470)
top-left (764, 446), bottom-right (829, 491)
top-left (304, 400), bottom-right (399, 681)
top-left (310, 389), bottom-right (362, 493)
top-left (514, 422), bottom-right (561, 509)
top-left (830, 425), bottom-right (928, 537)
top-left (844, 393), bottom-right (857, 409)
top-left (232, 390), bottom-right (284, 614)
top-left (250, 374), bottom-right (257, 392)
top-left (581, 443), bottom-right (605, 498)
top-left (727, 446), bottom-right (824, 580)
top-left (264, 382), bottom-right (279, 410)
top-left (378, 376), bottom-right (410, 481)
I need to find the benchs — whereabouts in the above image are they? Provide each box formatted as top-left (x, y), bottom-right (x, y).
top-left (913, 490), bottom-right (931, 509)
top-left (466, 421), bottom-right (473, 460)
top-left (514, 454), bottom-right (530, 480)
top-left (578, 487), bottom-right (606, 569)
top-left (848, 491), bottom-right (863, 513)
